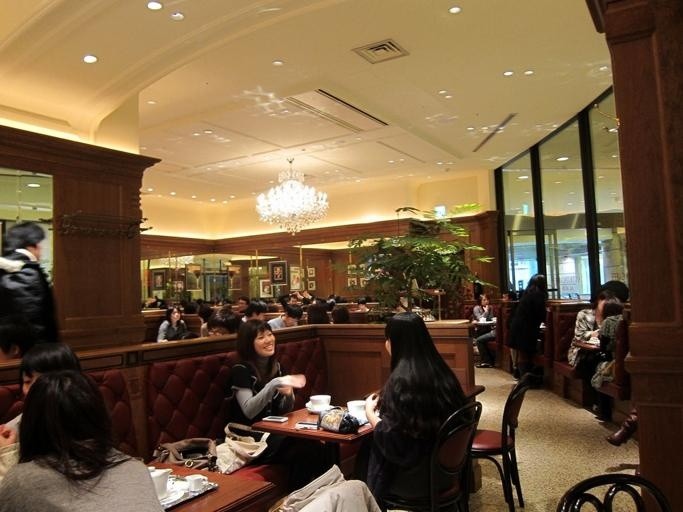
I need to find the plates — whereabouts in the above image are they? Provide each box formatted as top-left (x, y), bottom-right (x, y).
top-left (306, 405), bottom-right (333, 414)
top-left (160, 488), bottom-right (185, 506)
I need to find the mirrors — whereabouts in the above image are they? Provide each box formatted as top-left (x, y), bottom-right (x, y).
top-left (587, 92), bottom-right (632, 304)
top-left (0, 167), bottom-right (55, 289)
top-left (539, 118), bottom-right (592, 303)
top-left (496, 148), bottom-right (538, 307)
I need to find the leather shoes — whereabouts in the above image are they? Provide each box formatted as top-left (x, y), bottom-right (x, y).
top-left (476, 362), bottom-right (495, 368)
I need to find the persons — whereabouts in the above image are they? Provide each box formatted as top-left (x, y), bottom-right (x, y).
top-left (507, 274), bottom-right (547, 389)
top-left (0, 368), bottom-right (165, 512)
top-left (224, 319), bottom-right (307, 494)
top-left (0, 341), bottom-right (83, 462)
top-left (352, 312), bottom-right (467, 512)
top-left (358, 297), bottom-right (372, 310)
top-left (473, 293), bottom-right (518, 368)
top-left (397, 294), bottom-right (431, 315)
top-left (0, 221), bottom-right (61, 354)
top-left (148, 291), bottom-right (352, 343)
top-left (591, 297), bottom-right (629, 420)
top-left (567, 289), bottom-right (622, 414)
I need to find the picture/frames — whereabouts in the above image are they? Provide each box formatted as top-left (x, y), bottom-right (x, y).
top-left (307, 280), bottom-right (316, 291)
top-left (267, 260), bottom-right (288, 286)
top-left (151, 269), bottom-right (166, 291)
top-left (226, 265), bottom-right (242, 292)
top-left (259, 278), bottom-right (274, 298)
top-left (289, 264), bottom-right (303, 292)
top-left (307, 268), bottom-right (315, 278)
top-left (185, 262), bottom-right (203, 292)
top-left (346, 263), bottom-right (369, 289)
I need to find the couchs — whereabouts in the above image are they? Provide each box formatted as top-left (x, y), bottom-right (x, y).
top-left (462, 295), bottom-right (638, 443)
top-left (0, 367), bottom-right (136, 461)
top-left (142, 338), bottom-right (325, 485)
top-left (139, 298), bottom-right (392, 336)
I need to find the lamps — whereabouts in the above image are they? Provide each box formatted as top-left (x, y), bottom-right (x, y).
top-left (254, 156), bottom-right (329, 238)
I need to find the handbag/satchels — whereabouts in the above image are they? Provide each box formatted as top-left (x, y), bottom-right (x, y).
top-left (151, 422), bottom-right (270, 475)
top-left (318, 407), bottom-right (360, 434)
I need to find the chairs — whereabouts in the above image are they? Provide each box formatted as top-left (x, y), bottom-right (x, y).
top-left (555, 473), bottom-right (682, 512)
top-left (381, 401), bottom-right (482, 511)
top-left (467, 372), bottom-right (533, 511)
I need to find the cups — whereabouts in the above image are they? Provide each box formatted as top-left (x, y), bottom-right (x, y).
top-left (185, 474), bottom-right (208, 491)
top-left (347, 400), bottom-right (366, 414)
top-left (310, 395), bottom-right (331, 406)
top-left (148, 466), bottom-right (173, 500)
